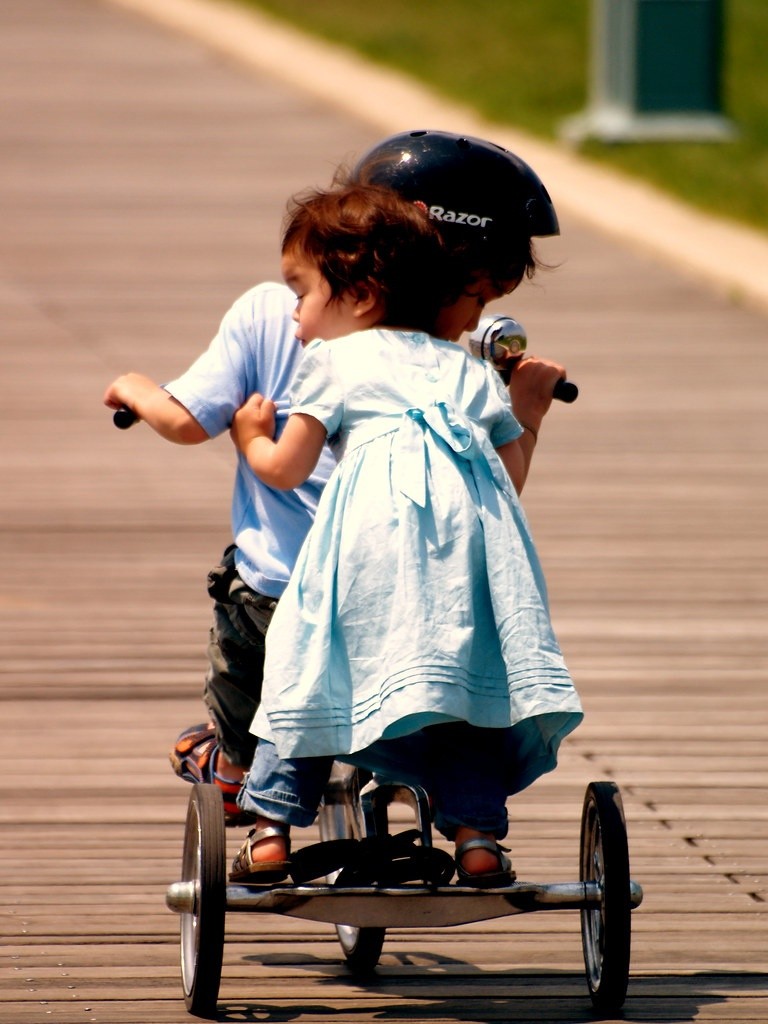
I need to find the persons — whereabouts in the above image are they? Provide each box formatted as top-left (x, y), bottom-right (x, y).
top-left (229, 184), bottom-right (584, 890)
top-left (104, 129), bottom-right (567, 813)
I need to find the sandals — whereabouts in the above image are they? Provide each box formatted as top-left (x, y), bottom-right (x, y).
top-left (168, 723), bottom-right (257, 827)
top-left (228, 826), bottom-right (294, 874)
top-left (455, 839), bottom-right (517, 887)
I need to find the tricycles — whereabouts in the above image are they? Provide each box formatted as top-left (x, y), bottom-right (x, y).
top-left (148, 311), bottom-right (647, 1024)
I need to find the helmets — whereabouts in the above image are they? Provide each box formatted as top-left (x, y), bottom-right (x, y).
top-left (355, 129), bottom-right (561, 236)
